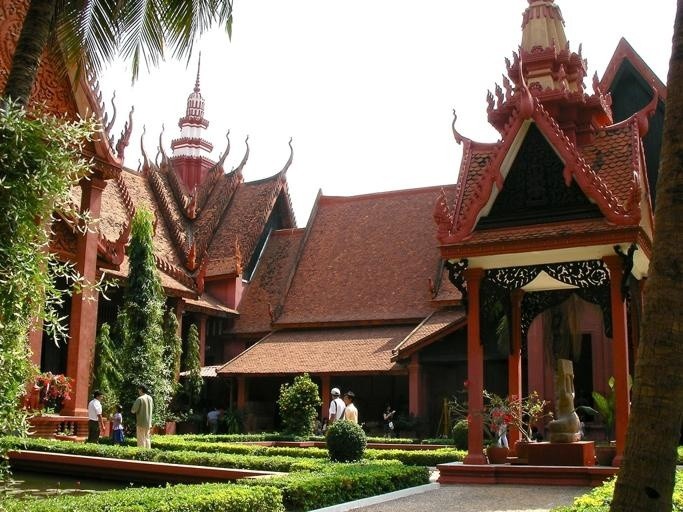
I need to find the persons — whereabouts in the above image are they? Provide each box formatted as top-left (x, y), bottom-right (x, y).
top-left (112, 405), bottom-right (125, 447)
top-left (207, 408), bottom-right (228, 434)
top-left (492, 409), bottom-right (511, 450)
top-left (88, 391), bottom-right (105, 444)
top-left (343, 391), bottom-right (359, 424)
top-left (383, 405), bottom-right (396, 425)
top-left (130, 385), bottom-right (152, 449)
top-left (329, 387), bottom-right (347, 423)
top-left (321, 418), bottom-right (328, 433)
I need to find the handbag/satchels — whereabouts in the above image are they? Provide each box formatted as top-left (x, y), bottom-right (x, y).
top-left (389, 422), bottom-right (395, 430)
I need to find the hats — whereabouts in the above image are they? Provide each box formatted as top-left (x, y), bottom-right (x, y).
top-left (116, 404), bottom-right (123, 409)
top-left (344, 391), bottom-right (355, 397)
top-left (330, 388), bottom-right (340, 396)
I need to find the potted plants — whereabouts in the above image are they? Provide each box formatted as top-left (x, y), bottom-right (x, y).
top-left (159, 412), bottom-right (178, 433)
top-left (444, 388), bottom-right (554, 465)
